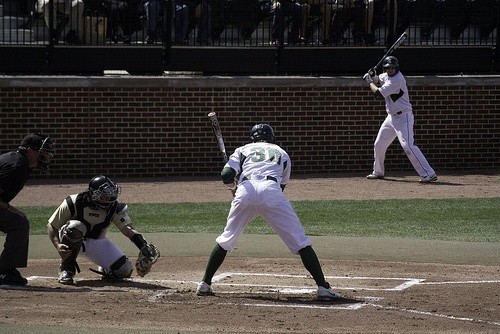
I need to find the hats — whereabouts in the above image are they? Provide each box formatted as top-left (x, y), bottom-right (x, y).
top-left (20, 134), bottom-right (43, 151)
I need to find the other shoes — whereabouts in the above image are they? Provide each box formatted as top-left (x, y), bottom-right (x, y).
top-left (0, 270), bottom-right (27, 286)
top-left (419, 174), bottom-right (437, 182)
top-left (276, 37), bottom-right (285, 48)
top-left (367, 174), bottom-right (384, 179)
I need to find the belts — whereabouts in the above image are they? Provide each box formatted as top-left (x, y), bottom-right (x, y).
top-left (393, 111), bottom-right (402, 116)
top-left (243, 175), bottom-right (277, 182)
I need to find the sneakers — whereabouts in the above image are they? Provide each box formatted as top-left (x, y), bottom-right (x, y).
top-left (197, 281), bottom-right (214, 294)
top-left (317, 282), bottom-right (340, 301)
top-left (98, 266), bottom-right (105, 280)
top-left (58, 270), bottom-right (74, 284)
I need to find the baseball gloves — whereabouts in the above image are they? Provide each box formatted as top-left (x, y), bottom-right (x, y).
top-left (136, 243), bottom-right (161, 278)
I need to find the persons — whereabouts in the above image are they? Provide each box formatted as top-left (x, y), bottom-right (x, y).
top-left (46, 175), bottom-right (161, 284)
top-left (0, 133), bottom-right (55, 286)
top-left (365, 56), bottom-right (437, 183)
top-left (24, 0), bottom-right (365, 45)
top-left (196, 123), bottom-right (339, 299)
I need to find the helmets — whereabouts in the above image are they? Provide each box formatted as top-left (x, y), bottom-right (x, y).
top-left (383, 56), bottom-right (399, 67)
top-left (89, 175), bottom-right (119, 207)
top-left (249, 124), bottom-right (276, 142)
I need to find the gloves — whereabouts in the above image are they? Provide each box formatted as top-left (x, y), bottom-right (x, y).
top-left (368, 68), bottom-right (378, 84)
top-left (362, 73), bottom-right (373, 83)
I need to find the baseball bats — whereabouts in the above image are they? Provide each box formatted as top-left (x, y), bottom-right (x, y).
top-left (208, 111), bottom-right (228, 163)
top-left (374, 32), bottom-right (409, 69)
